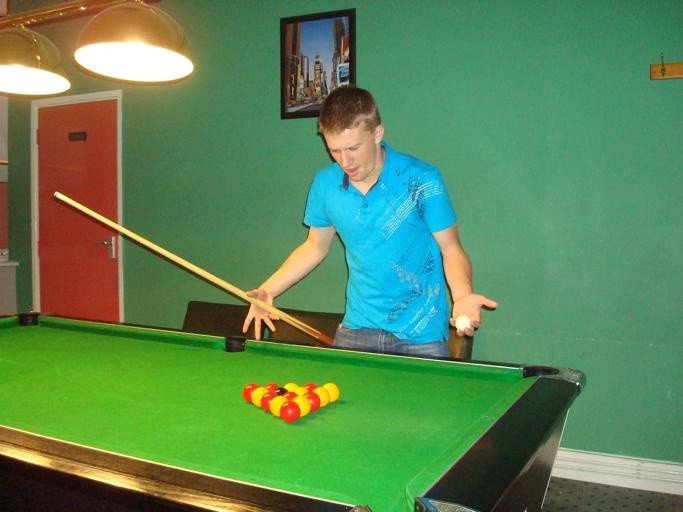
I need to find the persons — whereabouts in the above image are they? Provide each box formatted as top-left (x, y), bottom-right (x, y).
top-left (241, 86), bottom-right (500, 359)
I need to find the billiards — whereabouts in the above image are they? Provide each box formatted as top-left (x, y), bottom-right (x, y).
top-left (455, 315), bottom-right (472, 332)
top-left (243, 381), bottom-right (339, 420)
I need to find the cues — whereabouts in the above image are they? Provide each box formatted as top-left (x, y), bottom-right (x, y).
top-left (53, 190), bottom-right (333, 347)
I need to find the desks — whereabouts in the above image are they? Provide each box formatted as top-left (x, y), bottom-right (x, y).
top-left (0, 311), bottom-right (586, 512)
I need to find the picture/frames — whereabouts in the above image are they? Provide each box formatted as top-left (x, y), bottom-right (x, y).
top-left (280, 9), bottom-right (356, 119)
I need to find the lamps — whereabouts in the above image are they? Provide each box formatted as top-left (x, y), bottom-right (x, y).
top-left (0, 24), bottom-right (71, 96)
top-left (70, 1), bottom-right (196, 86)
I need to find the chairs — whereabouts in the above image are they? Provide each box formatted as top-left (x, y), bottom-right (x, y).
top-left (182, 301), bottom-right (266, 340)
top-left (448, 323), bottom-right (473, 360)
top-left (269, 307), bottom-right (345, 348)
